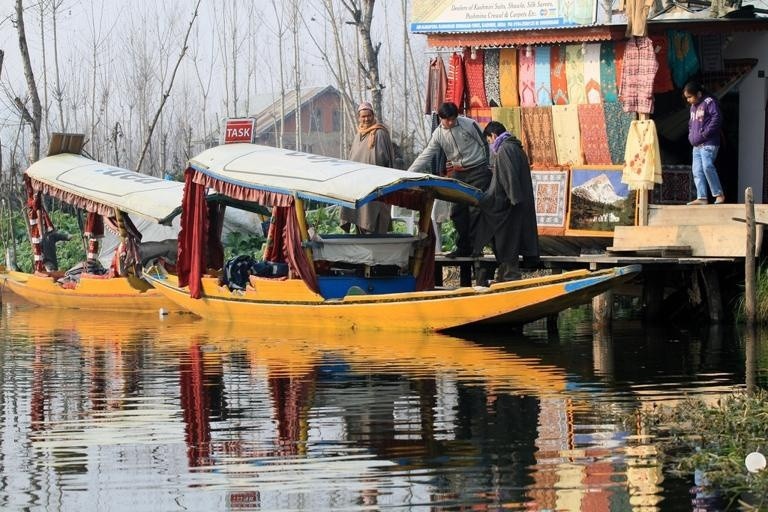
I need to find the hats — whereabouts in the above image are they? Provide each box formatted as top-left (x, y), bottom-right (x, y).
top-left (357, 101), bottom-right (372, 112)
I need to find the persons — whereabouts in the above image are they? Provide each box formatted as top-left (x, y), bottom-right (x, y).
top-left (683, 81), bottom-right (726, 205)
top-left (479, 121), bottom-right (541, 282)
top-left (341, 102), bottom-right (394, 234)
top-left (407, 102), bottom-right (492, 258)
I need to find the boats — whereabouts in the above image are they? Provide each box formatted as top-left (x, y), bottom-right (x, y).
top-left (137, 142), bottom-right (641, 334)
top-left (0, 156), bottom-right (216, 316)
